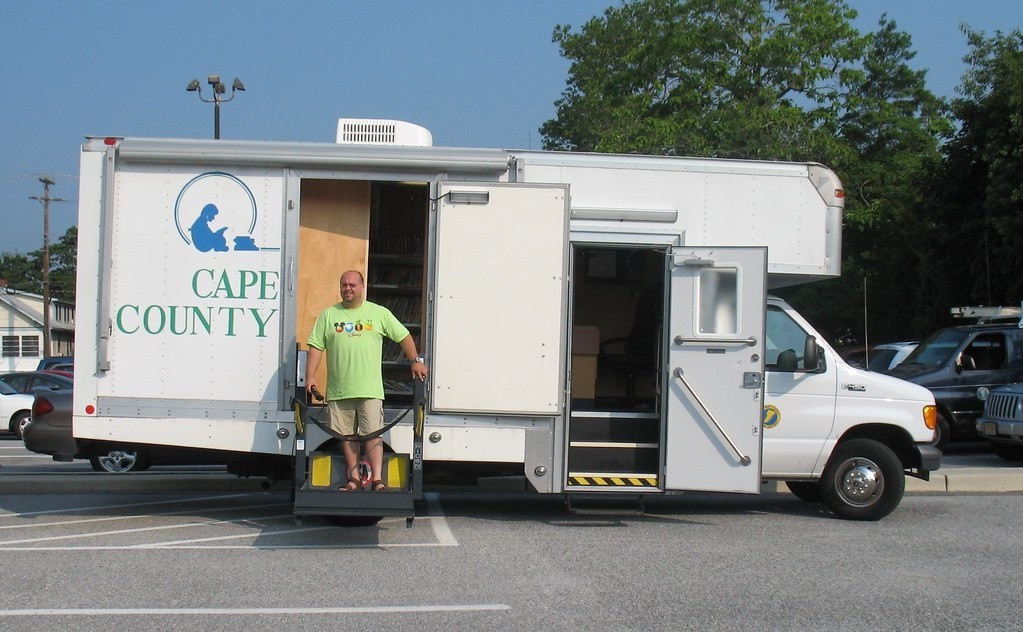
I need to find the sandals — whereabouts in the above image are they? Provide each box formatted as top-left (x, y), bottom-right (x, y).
top-left (339, 478), bottom-right (361, 491)
top-left (372, 480), bottom-right (390, 493)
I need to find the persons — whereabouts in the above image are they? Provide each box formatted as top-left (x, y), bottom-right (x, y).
top-left (304, 270), bottom-right (429, 492)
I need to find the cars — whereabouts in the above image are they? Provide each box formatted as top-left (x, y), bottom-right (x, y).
top-left (975, 384), bottom-right (1023, 461)
top-left (35, 356), bottom-right (75, 375)
top-left (0, 371), bottom-right (75, 438)
top-left (23, 386), bottom-right (168, 473)
top-left (858, 341), bottom-right (1000, 373)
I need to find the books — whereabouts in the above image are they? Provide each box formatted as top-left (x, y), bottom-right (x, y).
top-left (365, 233), bottom-right (424, 392)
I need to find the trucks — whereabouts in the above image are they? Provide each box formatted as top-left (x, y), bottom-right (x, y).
top-left (72, 119), bottom-right (942, 521)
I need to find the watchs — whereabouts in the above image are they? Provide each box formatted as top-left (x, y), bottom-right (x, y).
top-left (410, 356), bottom-right (421, 365)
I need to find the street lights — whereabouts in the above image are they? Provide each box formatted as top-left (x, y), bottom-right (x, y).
top-left (185, 74), bottom-right (246, 140)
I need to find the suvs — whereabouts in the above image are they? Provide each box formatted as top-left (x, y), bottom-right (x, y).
top-left (879, 305), bottom-right (1022, 452)
